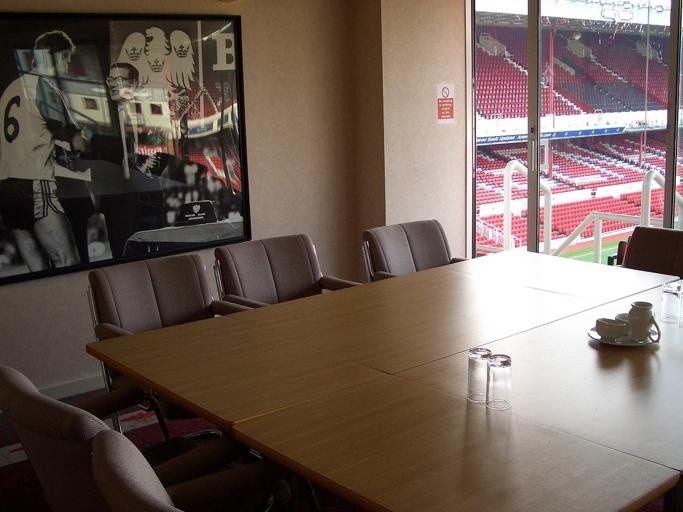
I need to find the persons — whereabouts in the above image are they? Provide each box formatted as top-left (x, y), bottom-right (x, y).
top-left (70, 63), bottom-right (169, 261)
top-left (0, 31), bottom-right (94, 273)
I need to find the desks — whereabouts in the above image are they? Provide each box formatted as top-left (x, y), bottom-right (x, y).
top-left (86, 250), bottom-right (683, 512)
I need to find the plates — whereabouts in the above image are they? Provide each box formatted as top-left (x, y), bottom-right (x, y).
top-left (587, 327), bottom-right (658, 347)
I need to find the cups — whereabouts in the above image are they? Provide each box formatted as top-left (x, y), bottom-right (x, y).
top-left (467, 348), bottom-right (514, 411)
top-left (661, 279), bottom-right (682, 323)
top-left (597, 301), bottom-right (662, 342)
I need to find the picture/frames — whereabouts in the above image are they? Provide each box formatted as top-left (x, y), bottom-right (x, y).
top-left (1, 9), bottom-right (252, 286)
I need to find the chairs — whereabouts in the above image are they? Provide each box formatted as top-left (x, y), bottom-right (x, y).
top-left (359, 219), bottom-right (470, 284)
top-left (212, 233), bottom-right (365, 308)
top-left (473, 11), bottom-right (683, 257)
top-left (607, 226), bottom-right (681, 278)
top-left (0, 363), bottom-right (356, 512)
top-left (82, 252), bottom-right (254, 439)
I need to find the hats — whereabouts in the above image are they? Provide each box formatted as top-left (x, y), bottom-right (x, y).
top-left (31, 31), bottom-right (72, 68)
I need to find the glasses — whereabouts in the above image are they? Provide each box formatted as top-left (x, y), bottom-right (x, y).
top-left (105, 74), bottom-right (134, 86)
